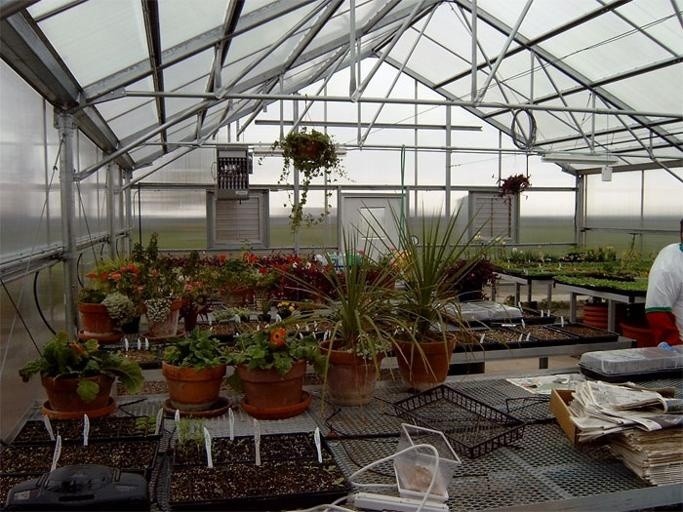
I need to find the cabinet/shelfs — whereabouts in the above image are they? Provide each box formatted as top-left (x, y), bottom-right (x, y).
top-left (0, 254), bottom-right (683, 512)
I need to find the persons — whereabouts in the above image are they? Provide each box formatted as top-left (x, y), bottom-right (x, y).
top-left (644, 217), bottom-right (682, 346)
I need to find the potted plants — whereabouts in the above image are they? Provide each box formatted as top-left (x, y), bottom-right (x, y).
top-left (284, 199), bottom-right (514, 412)
top-left (260, 125), bottom-right (348, 230)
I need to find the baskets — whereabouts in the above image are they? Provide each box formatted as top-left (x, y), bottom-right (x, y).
top-left (392, 379), bottom-right (525, 458)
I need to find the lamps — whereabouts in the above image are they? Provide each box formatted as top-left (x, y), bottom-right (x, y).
top-left (602, 115), bottom-right (612, 182)
